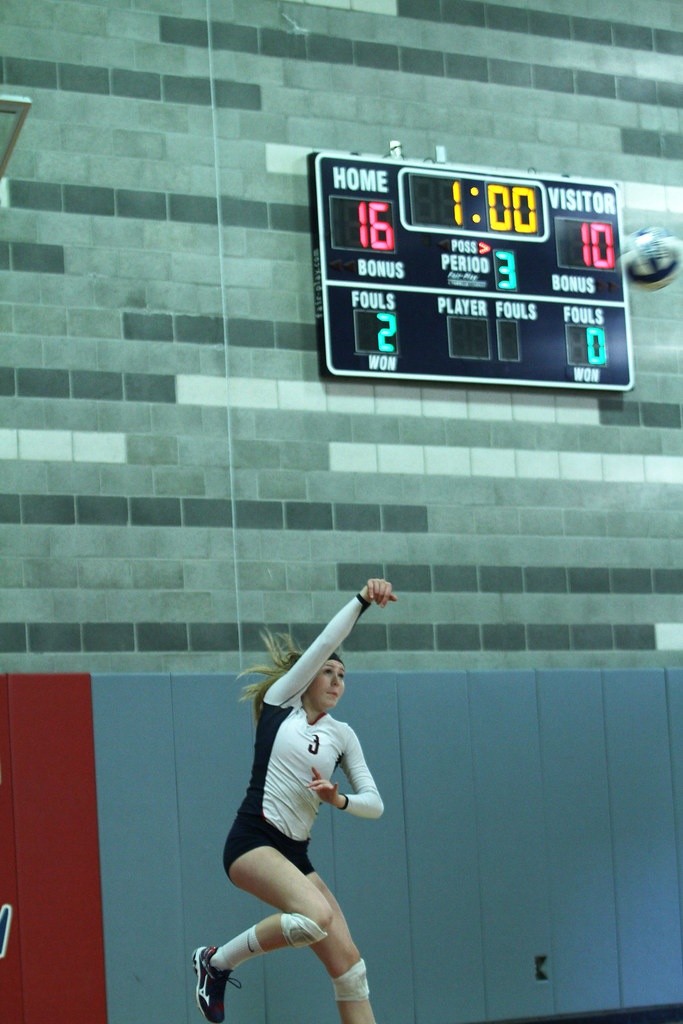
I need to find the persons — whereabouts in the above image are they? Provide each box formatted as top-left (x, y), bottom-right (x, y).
top-left (193, 578), bottom-right (398, 1024)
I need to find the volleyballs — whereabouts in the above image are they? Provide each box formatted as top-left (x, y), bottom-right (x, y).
top-left (620, 224), bottom-right (683, 293)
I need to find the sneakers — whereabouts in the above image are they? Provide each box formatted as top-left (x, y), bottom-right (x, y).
top-left (192, 946), bottom-right (241, 1024)
